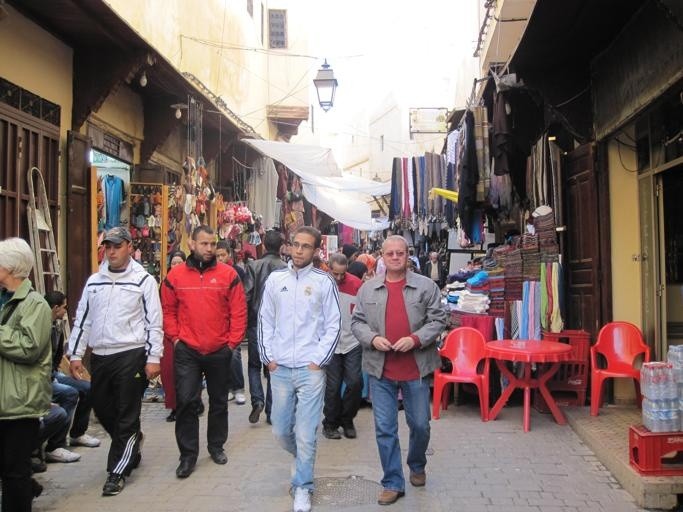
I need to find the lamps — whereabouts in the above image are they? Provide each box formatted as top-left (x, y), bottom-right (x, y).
top-left (170, 100), bottom-right (188, 119)
top-left (312, 55), bottom-right (338, 112)
top-left (140, 68), bottom-right (148, 87)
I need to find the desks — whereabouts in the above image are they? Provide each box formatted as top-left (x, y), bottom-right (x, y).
top-left (485, 340), bottom-right (575, 432)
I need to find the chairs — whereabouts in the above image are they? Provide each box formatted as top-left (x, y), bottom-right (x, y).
top-left (589, 321), bottom-right (651, 415)
top-left (433, 326), bottom-right (486, 422)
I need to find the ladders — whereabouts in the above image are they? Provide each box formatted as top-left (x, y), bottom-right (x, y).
top-left (28, 165), bottom-right (72, 356)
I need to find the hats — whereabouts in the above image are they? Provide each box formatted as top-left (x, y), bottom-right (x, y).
top-left (101, 226), bottom-right (132, 245)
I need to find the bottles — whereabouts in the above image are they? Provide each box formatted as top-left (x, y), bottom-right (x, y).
top-left (667, 344), bottom-right (683, 384)
top-left (640, 361), bottom-right (677, 400)
top-left (641, 397), bottom-right (680, 432)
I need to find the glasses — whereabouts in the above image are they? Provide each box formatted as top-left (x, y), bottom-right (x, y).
top-left (291, 242), bottom-right (316, 250)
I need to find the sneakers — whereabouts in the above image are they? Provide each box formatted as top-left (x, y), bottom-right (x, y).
top-left (46, 447), bottom-right (82, 462)
top-left (169, 391), bottom-right (273, 422)
top-left (103, 474), bottom-right (125, 495)
top-left (293, 487), bottom-right (312, 512)
top-left (70, 434), bottom-right (101, 447)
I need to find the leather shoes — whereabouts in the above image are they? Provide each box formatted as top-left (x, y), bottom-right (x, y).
top-left (409, 469), bottom-right (426, 486)
top-left (208, 448), bottom-right (227, 464)
top-left (342, 423), bottom-right (357, 439)
top-left (378, 490), bottom-right (404, 505)
top-left (176, 460), bottom-right (195, 477)
top-left (322, 428), bottom-right (340, 439)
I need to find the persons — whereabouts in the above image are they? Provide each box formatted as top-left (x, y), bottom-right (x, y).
top-left (155, 251), bottom-right (204, 423)
top-left (45, 289), bottom-right (101, 464)
top-left (352, 235), bottom-right (446, 507)
top-left (257, 228), bottom-right (340, 508)
top-left (211, 241), bottom-right (246, 405)
top-left (315, 242), bottom-right (381, 279)
top-left (244, 230), bottom-right (290, 427)
top-left (323, 253), bottom-right (364, 439)
top-left (1, 237), bottom-right (52, 511)
top-left (408, 243), bottom-right (445, 287)
top-left (28, 401), bottom-right (67, 473)
top-left (66, 226), bottom-right (164, 495)
top-left (164, 227), bottom-right (249, 474)
